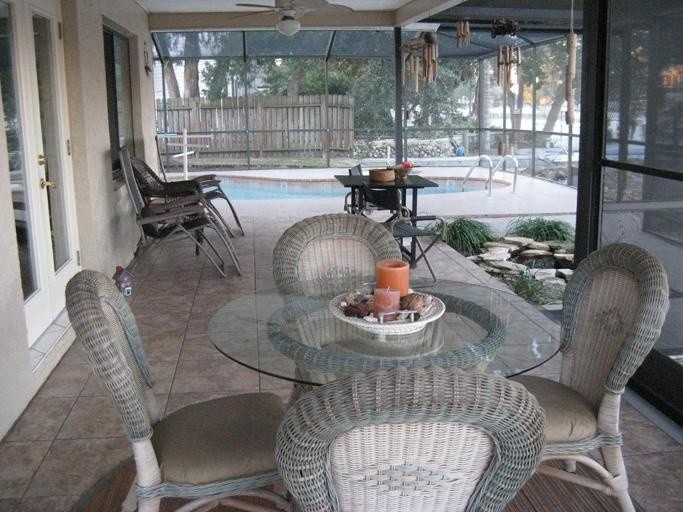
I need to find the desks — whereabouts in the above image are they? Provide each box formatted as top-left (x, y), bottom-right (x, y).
top-left (334, 174), bottom-right (440, 269)
top-left (211, 276), bottom-right (566, 444)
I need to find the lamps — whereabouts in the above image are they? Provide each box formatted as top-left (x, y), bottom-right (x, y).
top-left (276, 16), bottom-right (301, 37)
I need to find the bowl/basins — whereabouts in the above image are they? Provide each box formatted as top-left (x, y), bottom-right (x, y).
top-left (368, 169), bottom-right (395, 182)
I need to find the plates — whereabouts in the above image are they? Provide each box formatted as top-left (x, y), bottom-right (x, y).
top-left (327, 288), bottom-right (447, 336)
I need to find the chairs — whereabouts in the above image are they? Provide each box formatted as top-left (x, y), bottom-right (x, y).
top-left (67, 261), bottom-right (291, 512)
top-left (362, 184), bottom-right (445, 284)
top-left (112, 144), bottom-right (246, 285)
top-left (269, 212), bottom-right (407, 393)
top-left (271, 365), bottom-right (546, 512)
top-left (512, 242), bottom-right (671, 511)
top-left (344, 163), bottom-right (410, 215)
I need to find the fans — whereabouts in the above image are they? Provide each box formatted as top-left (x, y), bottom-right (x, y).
top-left (231, 0), bottom-right (315, 21)
